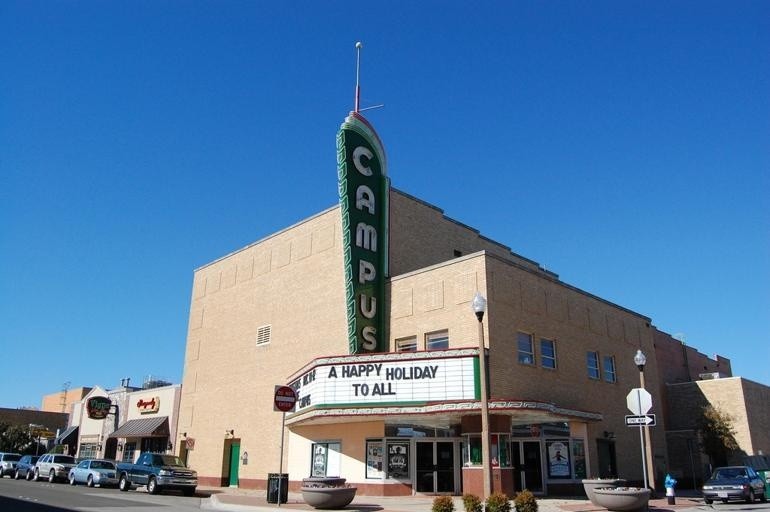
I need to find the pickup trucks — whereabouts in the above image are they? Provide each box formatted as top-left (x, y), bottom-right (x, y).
top-left (114, 452), bottom-right (198, 496)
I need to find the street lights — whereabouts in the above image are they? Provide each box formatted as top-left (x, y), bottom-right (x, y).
top-left (470, 290), bottom-right (493, 506)
top-left (633, 348), bottom-right (658, 500)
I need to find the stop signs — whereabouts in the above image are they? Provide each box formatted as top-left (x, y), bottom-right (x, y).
top-left (273, 386), bottom-right (296, 508)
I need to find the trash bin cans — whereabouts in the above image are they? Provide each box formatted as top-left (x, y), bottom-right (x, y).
top-left (267, 473), bottom-right (288, 504)
top-left (755, 469), bottom-right (770, 499)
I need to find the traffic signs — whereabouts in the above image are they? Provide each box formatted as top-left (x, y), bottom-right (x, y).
top-left (623, 413), bottom-right (656, 428)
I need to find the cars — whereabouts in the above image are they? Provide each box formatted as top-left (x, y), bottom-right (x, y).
top-left (14, 455), bottom-right (41, 480)
top-left (700, 465), bottom-right (767, 505)
top-left (0, 452), bottom-right (23, 478)
top-left (66, 458), bottom-right (115, 487)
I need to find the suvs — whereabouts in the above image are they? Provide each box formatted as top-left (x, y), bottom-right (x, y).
top-left (33, 453), bottom-right (78, 483)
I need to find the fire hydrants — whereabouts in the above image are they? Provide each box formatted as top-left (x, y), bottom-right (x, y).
top-left (664, 473), bottom-right (678, 505)
